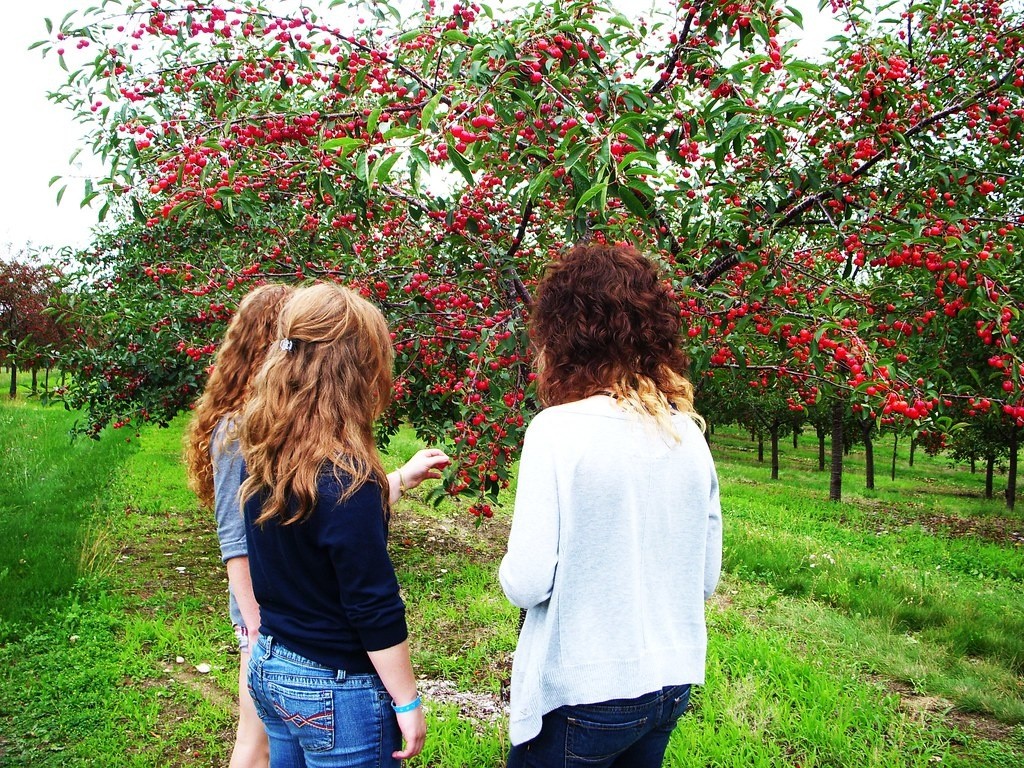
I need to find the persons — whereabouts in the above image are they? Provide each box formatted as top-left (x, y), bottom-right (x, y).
top-left (184, 284), bottom-right (452, 768)
top-left (495, 243), bottom-right (722, 768)
top-left (225, 284), bottom-right (427, 768)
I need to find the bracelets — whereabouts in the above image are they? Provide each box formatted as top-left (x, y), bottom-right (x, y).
top-left (391, 698), bottom-right (423, 713)
top-left (397, 468), bottom-right (406, 497)
